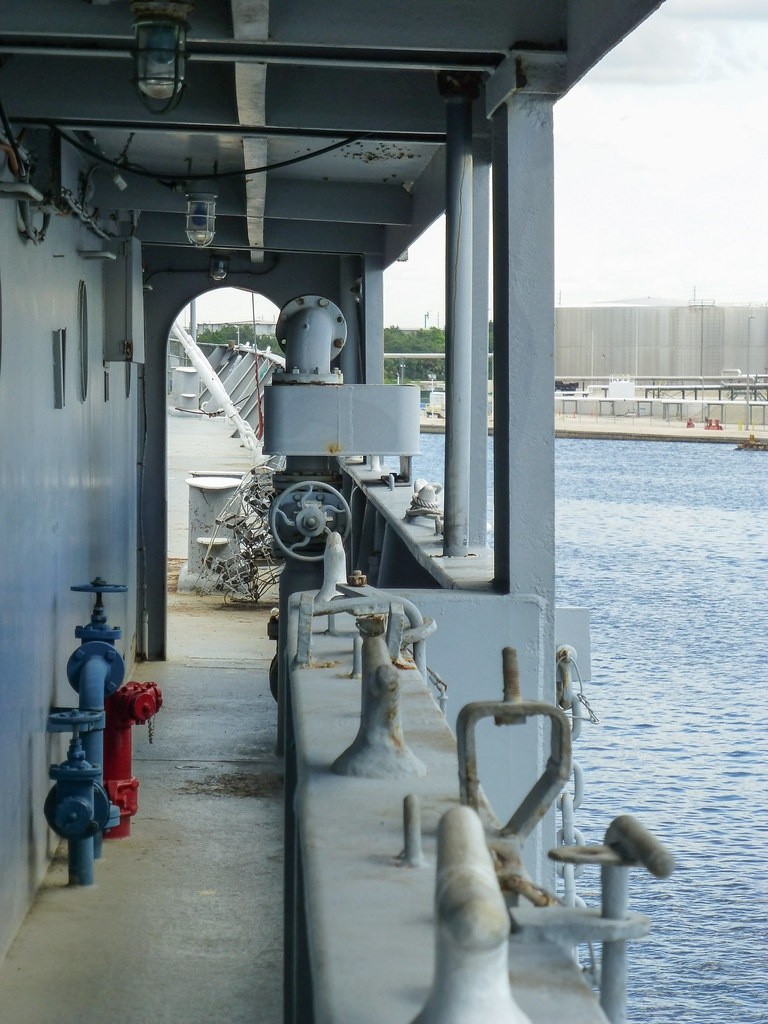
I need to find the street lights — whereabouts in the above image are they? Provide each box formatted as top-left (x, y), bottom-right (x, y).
top-left (747, 315), bottom-right (757, 432)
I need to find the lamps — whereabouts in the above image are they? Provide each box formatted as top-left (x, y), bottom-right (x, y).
top-left (183, 179), bottom-right (219, 249)
top-left (208, 253), bottom-right (230, 282)
top-left (130, 11), bottom-right (189, 117)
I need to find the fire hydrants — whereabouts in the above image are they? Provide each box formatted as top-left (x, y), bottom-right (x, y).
top-left (99, 681), bottom-right (163, 840)
